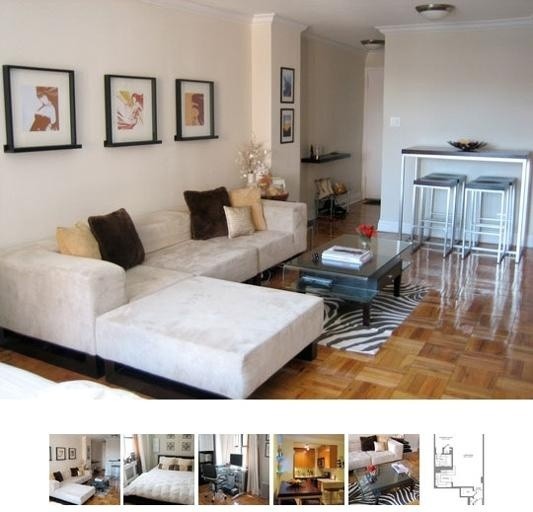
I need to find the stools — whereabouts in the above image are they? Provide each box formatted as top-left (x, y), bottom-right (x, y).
top-left (409, 172), bottom-right (518, 264)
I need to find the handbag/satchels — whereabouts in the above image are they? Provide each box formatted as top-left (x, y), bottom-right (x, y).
top-left (315, 177), bottom-right (334, 201)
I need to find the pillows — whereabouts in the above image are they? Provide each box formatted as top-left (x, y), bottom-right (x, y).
top-left (359, 435), bottom-right (386, 452)
top-left (56, 208), bottom-right (144, 270)
top-left (184, 185), bottom-right (265, 241)
top-left (51, 467), bottom-right (83, 483)
top-left (158, 455), bottom-right (194, 472)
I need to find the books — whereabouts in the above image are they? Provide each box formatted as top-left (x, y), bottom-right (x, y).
top-left (321, 244), bottom-right (372, 270)
top-left (391, 462), bottom-right (408, 473)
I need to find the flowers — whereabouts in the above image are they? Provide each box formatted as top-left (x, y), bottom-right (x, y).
top-left (236, 143), bottom-right (271, 177)
top-left (354, 223), bottom-right (375, 250)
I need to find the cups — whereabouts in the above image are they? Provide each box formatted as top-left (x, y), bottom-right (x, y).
top-left (313, 143), bottom-right (322, 160)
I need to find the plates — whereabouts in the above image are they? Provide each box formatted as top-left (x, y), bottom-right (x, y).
top-left (447, 140), bottom-right (487, 151)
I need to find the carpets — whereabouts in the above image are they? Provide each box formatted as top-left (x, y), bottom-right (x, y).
top-left (94, 486), bottom-right (113, 498)
top-left (349, 479), bottom-right (419, 504)
top-left (286, 279), bottom-right (431, 358)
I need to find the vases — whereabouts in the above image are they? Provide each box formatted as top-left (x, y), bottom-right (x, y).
top-left (247, 171), bottom-right (254, 187)
top-left (360, 239), bottom-right (371, 252)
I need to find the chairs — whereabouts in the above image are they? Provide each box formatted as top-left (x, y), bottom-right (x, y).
top-left (200, 461), bottom-right (229, 503)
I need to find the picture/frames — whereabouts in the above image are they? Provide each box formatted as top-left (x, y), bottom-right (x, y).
top-left (49, 447), bottom-right (76, 461)
top-left (103, 73), bottom-right (163, 148)
top-left (174, 78), bottom-right (218, 141)
top-left (2, 66), bottom-right (83, 154)
top-left (279, 65), bottom-right (295, 145)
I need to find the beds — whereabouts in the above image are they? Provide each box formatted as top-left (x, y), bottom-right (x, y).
top-left (125, 455), bottom-right (194, 505)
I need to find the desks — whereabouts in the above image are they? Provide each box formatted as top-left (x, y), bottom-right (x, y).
top-left (396, 145), bottom-right (530, 261)
top-left (276, 479), bottom-right (322, 505)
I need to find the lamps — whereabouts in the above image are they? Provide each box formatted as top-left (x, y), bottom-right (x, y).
top-left (360, 38), bottom-right (384, 51)
top-left (414, 4), bottom-right (455, 20)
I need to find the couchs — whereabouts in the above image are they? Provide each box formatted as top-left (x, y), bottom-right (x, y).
top-left (348, 434), bottom-right (404, 473)
top-left (49, 469), bottom-right (95, 505)
top-left (2, 197), bottom-right (326, 399)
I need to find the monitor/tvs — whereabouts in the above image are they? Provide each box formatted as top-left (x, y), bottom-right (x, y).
top-left (231, 454), bottom-right (242, 466)
